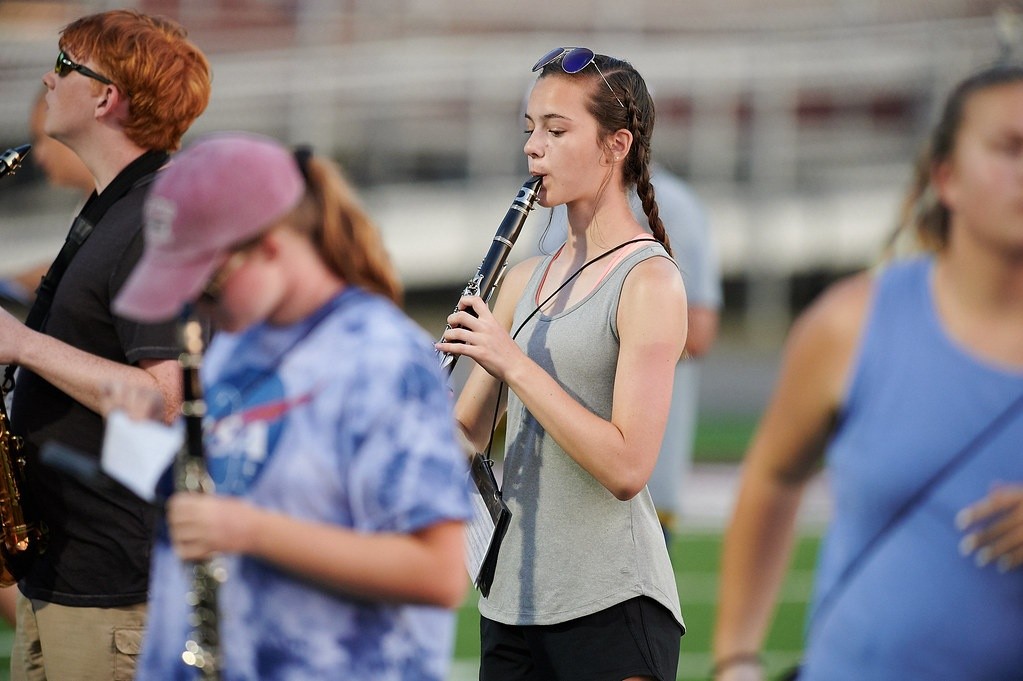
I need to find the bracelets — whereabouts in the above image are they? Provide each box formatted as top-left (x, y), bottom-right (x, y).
top-left (711, 654), bottom-right (760, 676)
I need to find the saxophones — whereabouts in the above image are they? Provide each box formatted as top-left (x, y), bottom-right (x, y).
top-left (1, 139), bottom-right (56, 591)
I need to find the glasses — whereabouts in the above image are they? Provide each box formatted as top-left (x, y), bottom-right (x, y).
top-left (532, 45), bottom-right (625, 108)
top-left (54, 50), bottom-right (122, 92)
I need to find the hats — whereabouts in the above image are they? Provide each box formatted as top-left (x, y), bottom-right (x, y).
top-left (112, 137), bottom-right (303, 323)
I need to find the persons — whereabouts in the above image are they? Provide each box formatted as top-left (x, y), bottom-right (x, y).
top-left (0, 10), bottom-right (212, 681)
top-left (433, 53), bottom-right (690, 681)
top-left (708, 66), bottom-right (1023, 681)
top-left (530, 160), bottom-right (724, 554)
top-left (109, 134), bottom-right (473, 681)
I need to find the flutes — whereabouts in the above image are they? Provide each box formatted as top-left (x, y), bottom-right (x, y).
top-left (173, 314), bottom-right (224, 681)
top-left (435, 173), bottom-right (549, 379)
